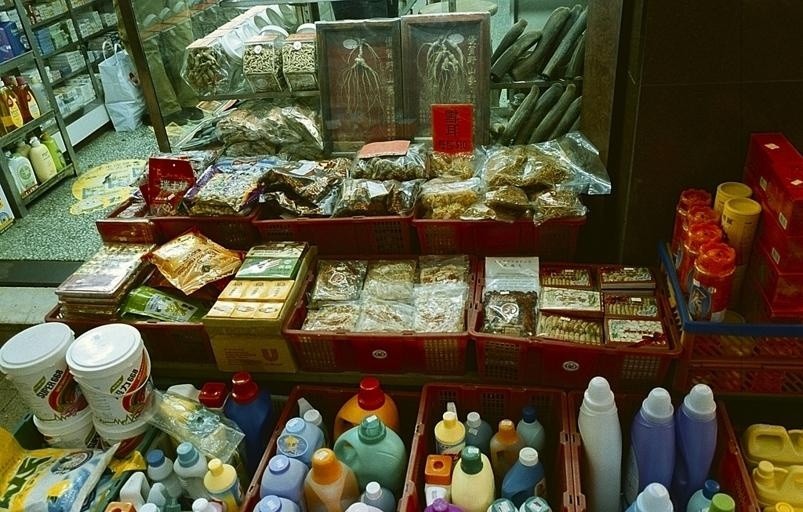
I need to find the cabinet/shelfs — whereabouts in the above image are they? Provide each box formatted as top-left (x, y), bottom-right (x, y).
top-left (0, 0), bottom-right (128, 236)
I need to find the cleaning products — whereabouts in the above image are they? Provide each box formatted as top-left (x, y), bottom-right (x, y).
top-left (251, 408), bottom-right (408, 512)
top-left (684, 479), bottom-right (720, 511)
top-left (103, 443), bottom-right (242, 512)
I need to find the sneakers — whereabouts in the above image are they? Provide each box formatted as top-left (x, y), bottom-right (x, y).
top-left (143, 106), bottom-right (205, 127)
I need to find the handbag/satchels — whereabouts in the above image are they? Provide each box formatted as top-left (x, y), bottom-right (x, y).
top-left (97, 48), bottom-right (150, 134)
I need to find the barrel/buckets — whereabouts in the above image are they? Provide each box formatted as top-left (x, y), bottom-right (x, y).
top-left (575, 376), bottom-right (623, 510)
top-left (738, 420), bottom-right (801, 512)
top-left (331, 377), bottom-right (402, 440)
top-left (0, 321), bottom-right (157, 461)
top-left (667, 384), bottom-right (718, 510)
top-left (625, 482), bottom-right (671, 512)
top-left (158, 381), bottom-right (228, 443)
top-left (421, 399), bottom-right (548, 511)
top-left (224, 372), bottom-right (276, 480)
top-left (624, 384), bottom-right (678, 504)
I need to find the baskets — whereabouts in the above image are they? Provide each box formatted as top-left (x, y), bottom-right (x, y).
top-left (282, 252), bottom-right (803, 396)
top-left (44, 304), bottom-right (215, 363)
top-left (11, 378), bottom-right (762, 512)
top-left (149, 203), bottom-right (587, 260)
